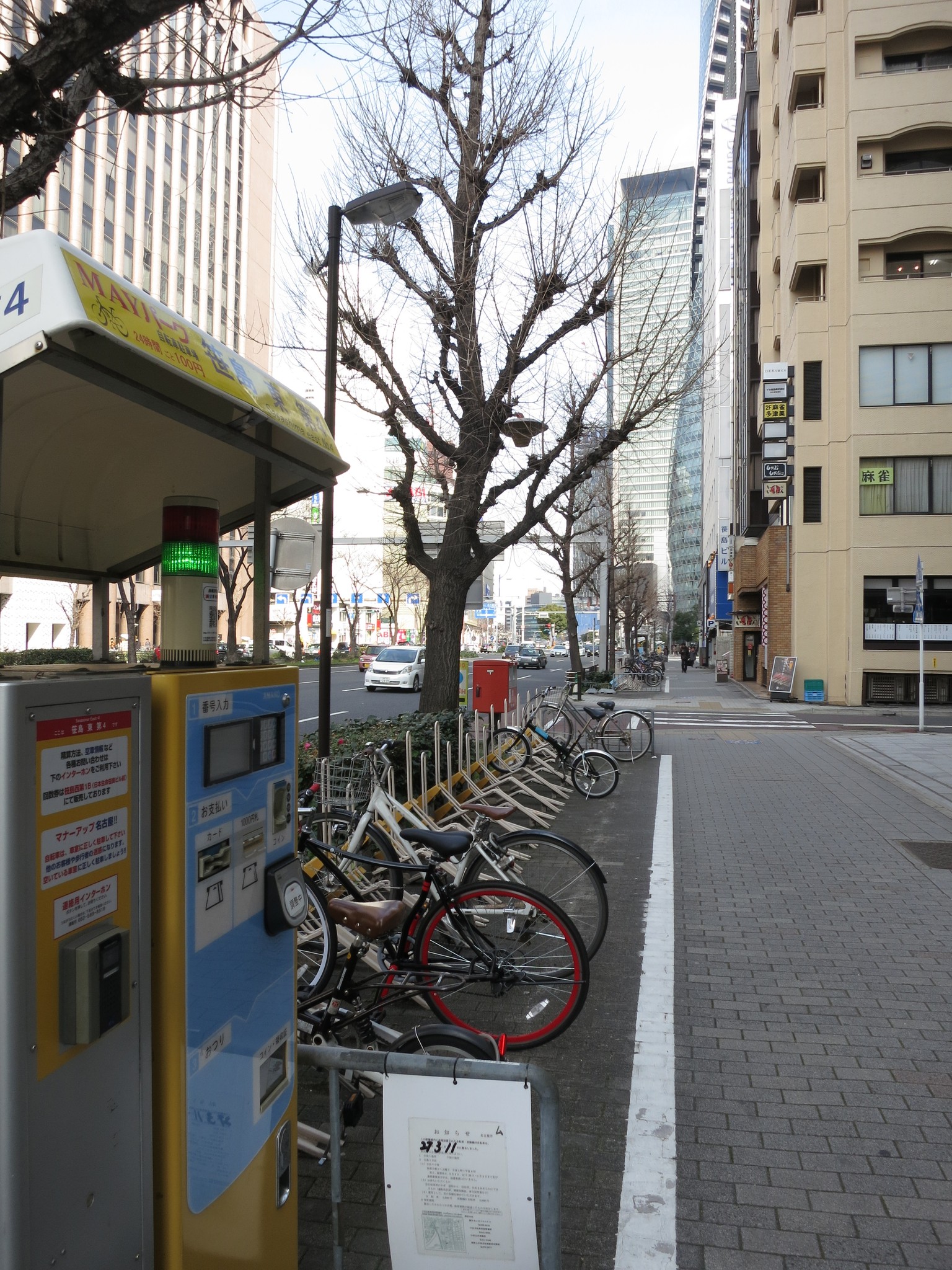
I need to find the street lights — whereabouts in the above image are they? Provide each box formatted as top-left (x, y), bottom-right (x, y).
top-left (316, 179), bottom-right (424, 756)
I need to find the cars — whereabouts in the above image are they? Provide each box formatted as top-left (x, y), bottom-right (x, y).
top-left (337, 642), bottom-right (359, 658)
top-left (302, 643), bottom-right (339, 658)
top-left (560, 638), bottom-right (618, 657)
top-left (515, 646), bottom-right (548, 670)
top-left (502, 645), bottom-right (524, 660)
top-left (550, 645), bottom-right (568, 658)
top-left (535, 643), bottom-right (545, 648)
top-left (364, 646), bottom-right (426, 693)
top-left (216, 642), bottom-right (281, 663)
top-left (359, 644), bottom-right (395, 672)
top-left (460, 641), bottom-right (512, 654)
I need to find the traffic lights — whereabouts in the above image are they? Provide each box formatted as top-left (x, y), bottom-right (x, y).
top-left (544, 624), bottom-right (551, 631)
top-left (371, 609), bottom-right (380, 613)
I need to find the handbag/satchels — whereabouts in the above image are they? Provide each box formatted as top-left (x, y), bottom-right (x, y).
top-left (681, 655), bottom-right (686, 660)
top-left (114, 645), bottom-right (118, 649)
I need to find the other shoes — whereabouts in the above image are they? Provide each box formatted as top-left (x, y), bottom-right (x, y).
top-left (682, 669), bottom-right (684, 672)
top-left (684, 671), bottom-right (686, 673)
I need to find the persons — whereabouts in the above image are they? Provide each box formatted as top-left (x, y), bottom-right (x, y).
top-left (134, 635), bottom-right (141, 651)
top-left (523, 651), bottom-right (529, 655)
top-left (662, 642), bottom-right (696, 673)
top-left (145, 638), bottom-right (151, 651)
top-left (110, 638), bottom-right (118, 651)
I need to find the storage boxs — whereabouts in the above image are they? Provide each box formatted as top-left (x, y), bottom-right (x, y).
top-left (804, 690), bottom-right (824, 702)
top-left (804, 679), bottom-right (823, 690)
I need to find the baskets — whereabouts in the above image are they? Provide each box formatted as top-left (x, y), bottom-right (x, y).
top-left (625, 657), bottom-right (631, 665)
top-left (313, 756), bottom-right (385, 807)
top-left (542, 686), bottom-right (564, 704)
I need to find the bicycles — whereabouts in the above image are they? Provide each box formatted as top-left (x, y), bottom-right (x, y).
top-left (519, 683), bottom-right (653, 765)
top-left (296, 738), bottom-right (610, 1141)
top-left (486, 685), bottom-right (621, 802)
top-left (620, 651), bottom-right (667, 687)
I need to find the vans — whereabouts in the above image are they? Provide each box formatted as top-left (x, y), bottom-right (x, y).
top-left (269, 638), bottom-right (296, 660)
top-left (521, 640), bottom-right (537, 655)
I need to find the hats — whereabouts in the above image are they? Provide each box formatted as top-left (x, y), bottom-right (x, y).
top-left (680, 643), bottom-right (686, 646)
